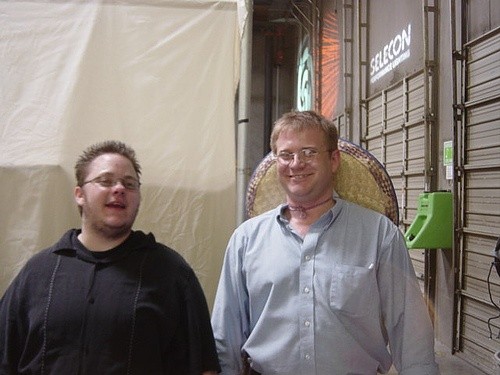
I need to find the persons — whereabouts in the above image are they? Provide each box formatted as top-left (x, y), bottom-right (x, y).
top-left (209, 109), bottom-right (440, 375)
top-left (0, 140), bottom-right (221, 375)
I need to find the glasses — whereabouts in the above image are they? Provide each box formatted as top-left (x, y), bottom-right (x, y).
top-left (81, 173), bottom-right (141, 191)
top-left (273, 148), bottom-right (333, 166)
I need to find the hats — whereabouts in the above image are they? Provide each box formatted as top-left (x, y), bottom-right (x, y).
top-left (246, 138), bottom-right (399, 228)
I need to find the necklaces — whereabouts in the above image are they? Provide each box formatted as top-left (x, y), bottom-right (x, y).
top-left (285, 198), bottom-right (333, 220)
